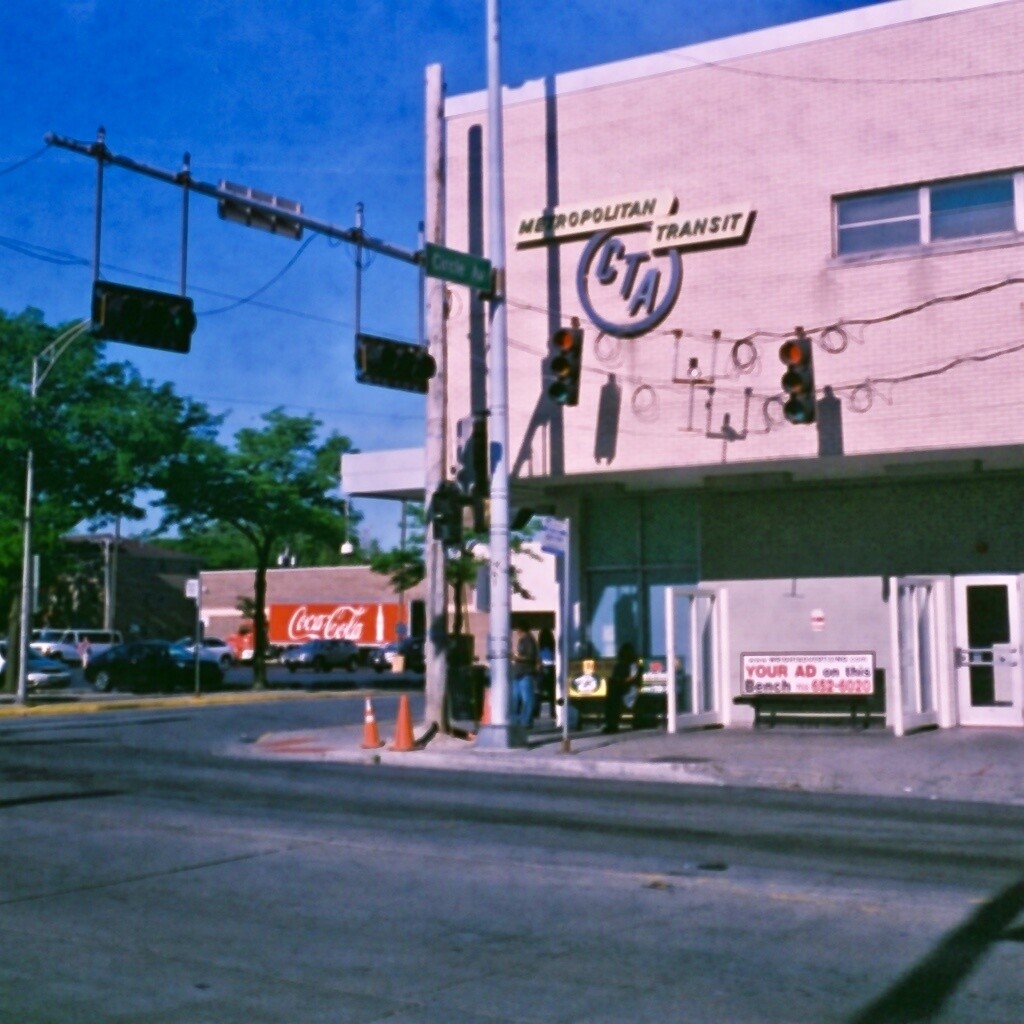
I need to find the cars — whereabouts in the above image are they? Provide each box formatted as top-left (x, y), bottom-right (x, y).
top-left (83, 636), bottom-right (235, 694)
top-left (239, 639), bottom-right (405, 675)
top-left (0, 640), bottom-right (71, 693)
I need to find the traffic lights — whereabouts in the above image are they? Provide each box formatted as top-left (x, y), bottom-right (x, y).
top-left (89, 282), bottom-right (195, 354)
top-left (546, 329), bottom-right (583, 406)
top-left (353, 333), bottom-right (437, 395)
top-left (778, 339), bottom-right (814, 424)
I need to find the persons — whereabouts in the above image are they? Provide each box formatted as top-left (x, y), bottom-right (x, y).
top-left (533, 620), bottom-right (556, 721)
top-left (512, 617), bottom-right (536, 728)
top-left (602, 643), bottom-right (640, 734)
top-left (77, 636), bottom-right (89, 668)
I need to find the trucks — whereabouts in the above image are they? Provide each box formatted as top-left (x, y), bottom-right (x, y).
top-left (223, 603), bottom-right (409, 665)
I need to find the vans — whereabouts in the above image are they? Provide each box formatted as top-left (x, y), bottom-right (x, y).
top-left (2, 626), bottom-right (124, 668)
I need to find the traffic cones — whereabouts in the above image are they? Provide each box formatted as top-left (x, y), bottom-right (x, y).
top-left (360, 696), bottom-right (381, 749)
top-left (480, 689), bottom-right (489, 726)
top-left (386, 694), bottom-right (421, 750)
